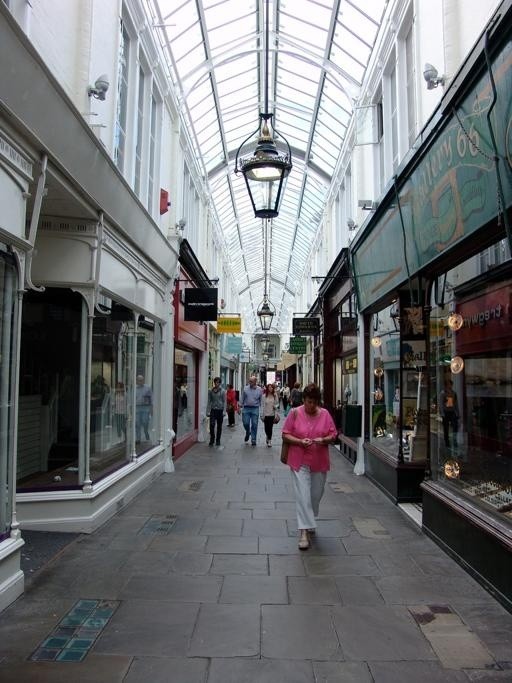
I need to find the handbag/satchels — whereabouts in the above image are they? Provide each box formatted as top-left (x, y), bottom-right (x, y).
top-left (273, 415), bottom-right (280, 424)
top-left (281, 440), bottom-right (290, 464)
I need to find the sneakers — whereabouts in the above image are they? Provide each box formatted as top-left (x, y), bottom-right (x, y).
top-left (266, 438), bottom-right (271, 447)
top-left (209, 438), bottom-right (220, 445)
top-left (245, 436), bottom-right (248, 441)
top-left (252, 443), bottom-right (256, 445)
top-left (298, 534), bottom-right (309, 548)
top-left (308, 527), bottom-right (316, 533)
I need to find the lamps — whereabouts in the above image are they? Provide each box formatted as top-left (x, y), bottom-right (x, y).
top-left (257, 219), bottom-right (276, 330)
top-left (89, 74), bottom-right (109, 102)
top-left (421, 64), bottom-right (445, 89)
top-left (175, 218), bottom-right (186, 230)
top-left (233, 0), bottom-right (292, 218)
top-left (347, 217), bottom-right (358, 231)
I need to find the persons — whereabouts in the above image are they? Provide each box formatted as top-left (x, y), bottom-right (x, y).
top-left (224, 381), bottom-right (304, 429)
top-left (113, 381), bottom-right (129, 444)
top-left (238, 376), bottom-right (263, 445)
top-left (439, 378), bottom-right (461, 448)
top-left (281, 384), bottom-right (339, 550)
top-left (135, 374), bottom-right (153, 443)
top-left (259, 383), bottom-right (279, 447)
top-left (206, 376), bottom-right (228, 446)
